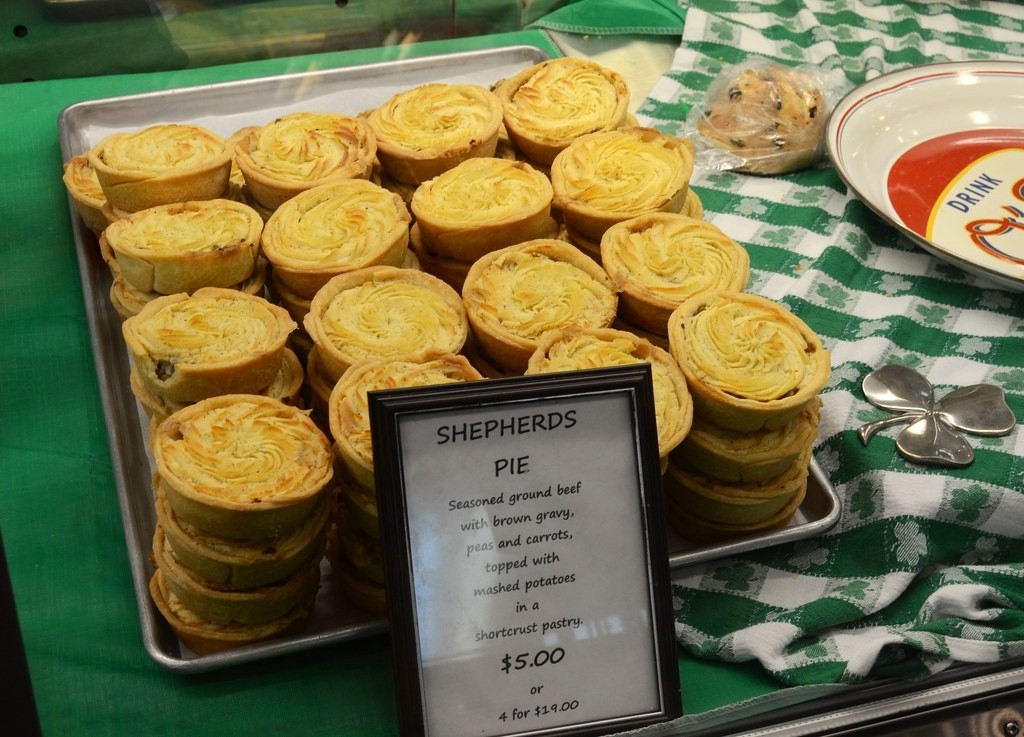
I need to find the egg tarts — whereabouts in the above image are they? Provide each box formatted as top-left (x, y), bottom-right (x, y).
top-left (65, 56), bottom-right (831, 657)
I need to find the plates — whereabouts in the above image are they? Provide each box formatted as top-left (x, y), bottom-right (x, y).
top-left (823, 61), bottom-right (1024, 291)
